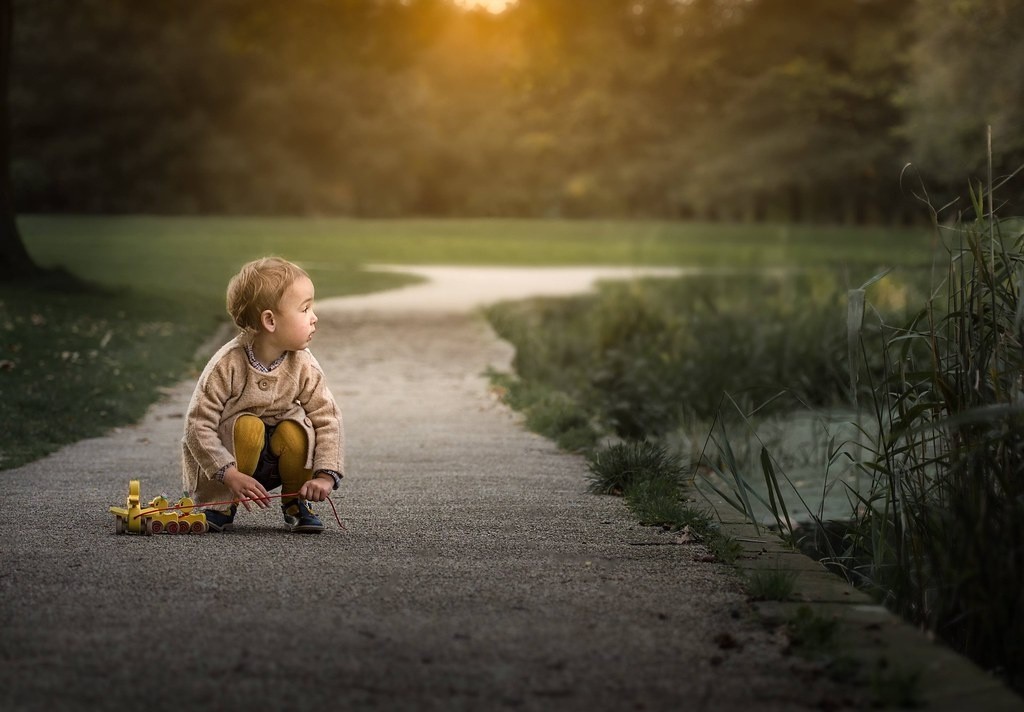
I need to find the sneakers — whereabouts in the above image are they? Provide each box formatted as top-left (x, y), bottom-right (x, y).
top-left (203, 503), bottom-right (236, 530)
top-left (281, 497), bottom-right (322, 532)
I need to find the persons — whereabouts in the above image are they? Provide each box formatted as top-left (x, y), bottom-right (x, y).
top-left (181, 254), bottom-right (344, 534)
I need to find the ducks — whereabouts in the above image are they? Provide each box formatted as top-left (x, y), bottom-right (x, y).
top-left (147, 496), bottom-right (180, 534)
top-left (174, 496), bottom-right (210, 534)
